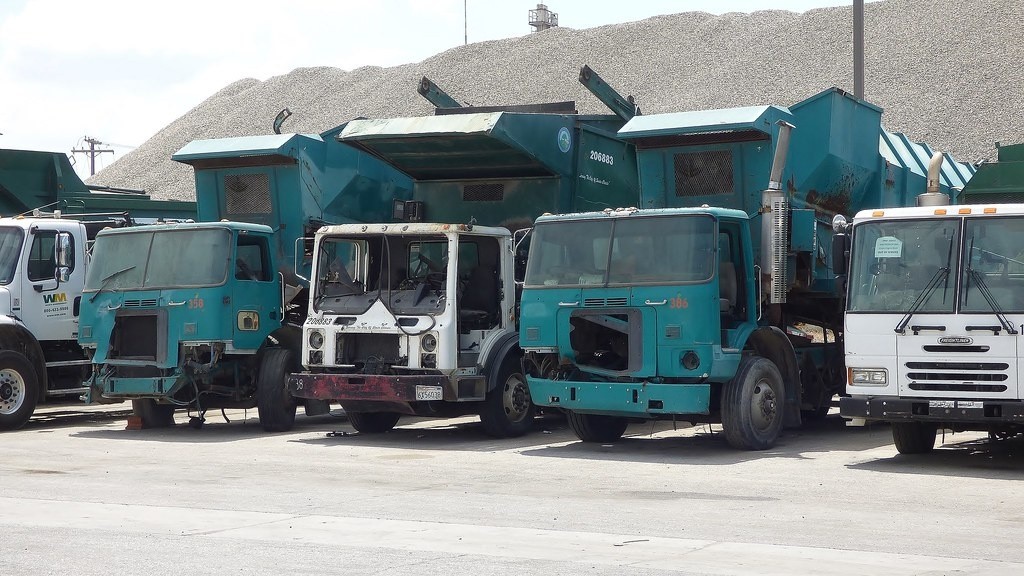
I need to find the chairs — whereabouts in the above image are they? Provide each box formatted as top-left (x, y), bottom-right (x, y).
top-left (700, 260), bottom-right (737, 317)
top-left (904, 264), bottom-right (936, 289)
top-left (603, 255), bottom-right (637, 276)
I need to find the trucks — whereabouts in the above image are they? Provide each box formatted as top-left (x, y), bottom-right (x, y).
top-left (510, 86), bottom-right (982, 443)
top-left (1, 64), bottom-right (639, 435)
top-left (833, 139), bottom-right (1023, 455)
